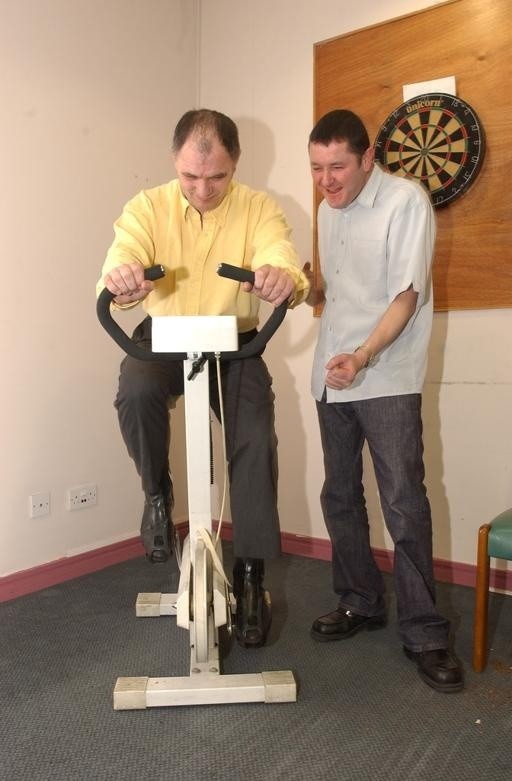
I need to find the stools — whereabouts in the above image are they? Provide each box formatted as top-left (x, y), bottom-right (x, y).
top-left (471, 509), bottom-right (511, 676)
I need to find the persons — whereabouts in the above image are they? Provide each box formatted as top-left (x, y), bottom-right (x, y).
top-left (303, 108), bottom-right (464, 691)
top-left (94, 110), bottom-right (310, 649)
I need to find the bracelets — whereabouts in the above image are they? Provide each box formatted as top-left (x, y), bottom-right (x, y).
top-left (353, 346), bottom-right (374, 367)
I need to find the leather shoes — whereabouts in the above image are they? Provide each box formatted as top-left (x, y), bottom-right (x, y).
top-left (141, 477), bottom-right (176, 565)
top-left (233, 557), bottom-right (271, 649)
top-left (310, 607), bottom-right (386, 642)
top-left (403, 645), bottom-right (464, 694)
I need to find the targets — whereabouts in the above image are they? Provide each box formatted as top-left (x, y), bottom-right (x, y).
top-left (373, 93), bottom-right (486, 210)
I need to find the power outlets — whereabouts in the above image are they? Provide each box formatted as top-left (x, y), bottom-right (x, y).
top-left (67, 483), bottom-right (99, 512)
top-left (30, 492), bottom-right (51, 517)
